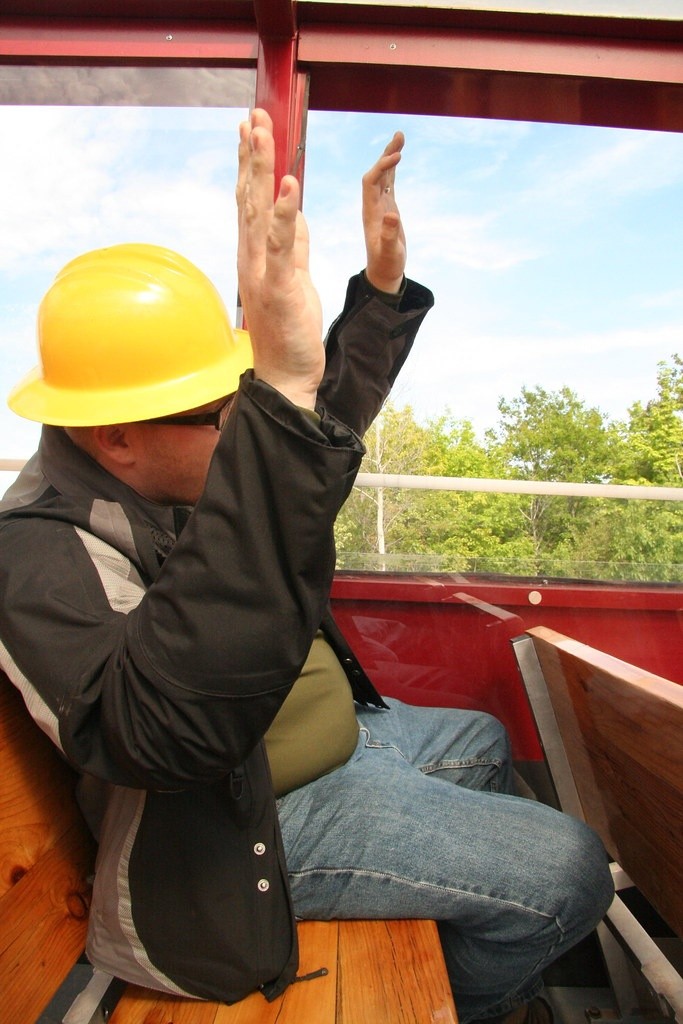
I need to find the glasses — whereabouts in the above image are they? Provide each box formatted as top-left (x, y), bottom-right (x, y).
top-left (142, 390), bottom-right (241, 430)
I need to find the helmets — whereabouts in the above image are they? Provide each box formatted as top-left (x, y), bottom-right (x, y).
top-left (11, 244), bottom-right (255, 427)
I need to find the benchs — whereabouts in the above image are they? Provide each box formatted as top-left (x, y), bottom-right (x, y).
top-left (0, 666), bottom-right (458, 1024)
top-left (513, 625), bottom-right (683, 1024)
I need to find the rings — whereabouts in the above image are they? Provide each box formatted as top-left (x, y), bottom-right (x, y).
top-left (385, 187), bottom-right (390, 193)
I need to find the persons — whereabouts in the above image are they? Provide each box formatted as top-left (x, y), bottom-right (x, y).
top-left (0, 109), bottom-right (615, 1024)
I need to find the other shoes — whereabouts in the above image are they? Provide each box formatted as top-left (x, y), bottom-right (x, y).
top-left (486, 998), bottom-right (555, 1024)
